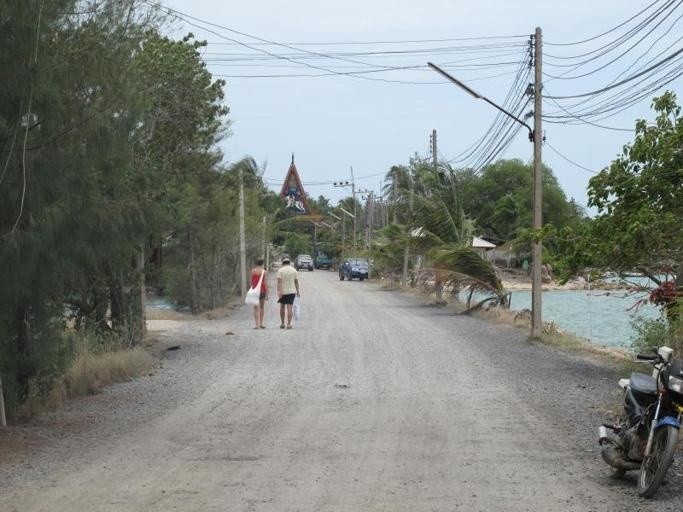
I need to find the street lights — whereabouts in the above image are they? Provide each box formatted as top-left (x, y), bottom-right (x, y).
top-left (427, 26), bottom-right (546, 341)
top-left (311, 166), bottom-right (357, 253)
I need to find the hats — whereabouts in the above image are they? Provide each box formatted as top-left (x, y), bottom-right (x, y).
top-left (282, 258), bottom-right (290, 264)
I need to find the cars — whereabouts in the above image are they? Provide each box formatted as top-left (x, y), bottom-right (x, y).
top-left (339, 258), bottom-right (368, 281)
top-left (294, 255), bottom-right (330, 271)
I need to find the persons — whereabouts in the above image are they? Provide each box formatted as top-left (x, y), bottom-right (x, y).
top-left (248, 257), bottom-right (270, 330)
top-left (276, 257), bottom-right (301, 330)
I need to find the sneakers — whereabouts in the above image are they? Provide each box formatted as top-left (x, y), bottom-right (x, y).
top-left (280, 325), bottom-right (285, 329)
top-left (287, 325), bottom-right (292, 329)
top-left (252, 325), bottom-right (266, 329)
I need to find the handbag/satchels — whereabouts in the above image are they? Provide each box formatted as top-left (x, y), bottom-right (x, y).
top-left (292, 295), bottom-right (301, 323)
top-left (244, 286), bottom-right (262, 306)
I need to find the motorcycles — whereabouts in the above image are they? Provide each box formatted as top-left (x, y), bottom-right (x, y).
top-left (597, 346), bottom-right (683, 499)
